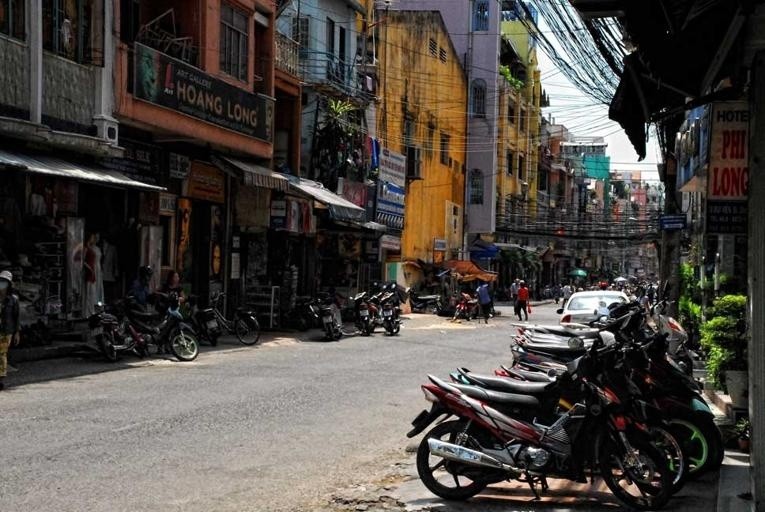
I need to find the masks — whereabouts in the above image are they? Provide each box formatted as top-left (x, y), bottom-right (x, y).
top-left (0, 281), bottom-right (9, 290)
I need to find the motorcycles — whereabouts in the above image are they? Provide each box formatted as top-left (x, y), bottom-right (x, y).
top-left (89, 286), bottom-right (222, 362)
top-left (304, 279), bottom-right (400, 341)
top-left (408, 288), bottom-right (441, 313)
top-left (451, 294), bottom-right (479, 322)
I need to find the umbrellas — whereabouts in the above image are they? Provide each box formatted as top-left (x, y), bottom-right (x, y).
top-left (568, 270), bottom-right (587, 277)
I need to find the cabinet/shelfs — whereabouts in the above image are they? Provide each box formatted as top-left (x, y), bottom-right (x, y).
top-left (245, 285), bottom-right (280, 329)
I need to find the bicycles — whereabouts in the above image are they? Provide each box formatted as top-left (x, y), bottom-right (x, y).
top-left (211, 290), bottom-right (260, 346)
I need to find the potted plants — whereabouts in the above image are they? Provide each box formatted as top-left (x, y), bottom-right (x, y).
top-left (699, 295), bottom-right (749, 408)
top-left (733, 418), bottom-right (752, 449)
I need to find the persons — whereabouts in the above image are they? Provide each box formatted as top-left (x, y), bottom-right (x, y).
top-left (474, 282), bottom-right (494, 324)
top-left (89, 234), bottom-right (105, 305)
top-left (126, 265), bottom-right (153, 309)
top-left (210, 244), bottom-right (221, 275)
top-left (548, 276), bottom-right (670, 310)
top-left (515, 280), bottom-right (529, 321)
top-left (0, 271), bottom-right (21, 391)
top-left (71, 232), bottom-right (96, 316)
top-left (210, 206), bottom-right (220, 240)
top-left (509, 278), bottom-right (521, 304)
top-left (175, 198), bottom-right (192, 270)
top-left (154, 272), bottom-right (185, 353)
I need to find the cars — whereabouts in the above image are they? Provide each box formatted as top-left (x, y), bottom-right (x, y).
top-left (558, 289), bottom-right (628, 325)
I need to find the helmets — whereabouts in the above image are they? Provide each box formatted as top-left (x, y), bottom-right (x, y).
top-left (139, 265), bottom-right (154, 275)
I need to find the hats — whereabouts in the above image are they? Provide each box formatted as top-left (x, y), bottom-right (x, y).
top-left (0, 270), bottom-right (12, 282)
